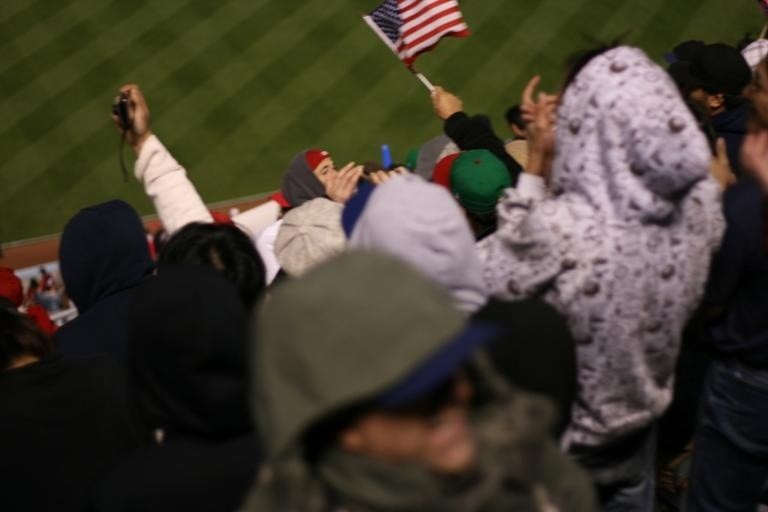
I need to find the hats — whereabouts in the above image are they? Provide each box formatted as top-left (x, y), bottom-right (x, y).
top-left (303, 148), bottom-right (332, 173)
top-left (669, 43), bottom-right (752, 91)
top-left (429, 146), bottom-right (515, 215)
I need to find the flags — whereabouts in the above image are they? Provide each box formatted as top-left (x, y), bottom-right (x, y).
top-left (360, 0), bottom-right (472, 66)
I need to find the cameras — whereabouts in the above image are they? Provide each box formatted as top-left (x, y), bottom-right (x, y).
top-left (354, 171), bottom-right (369, 190)
top-left (111, 90), bottom-right (135, 131)
